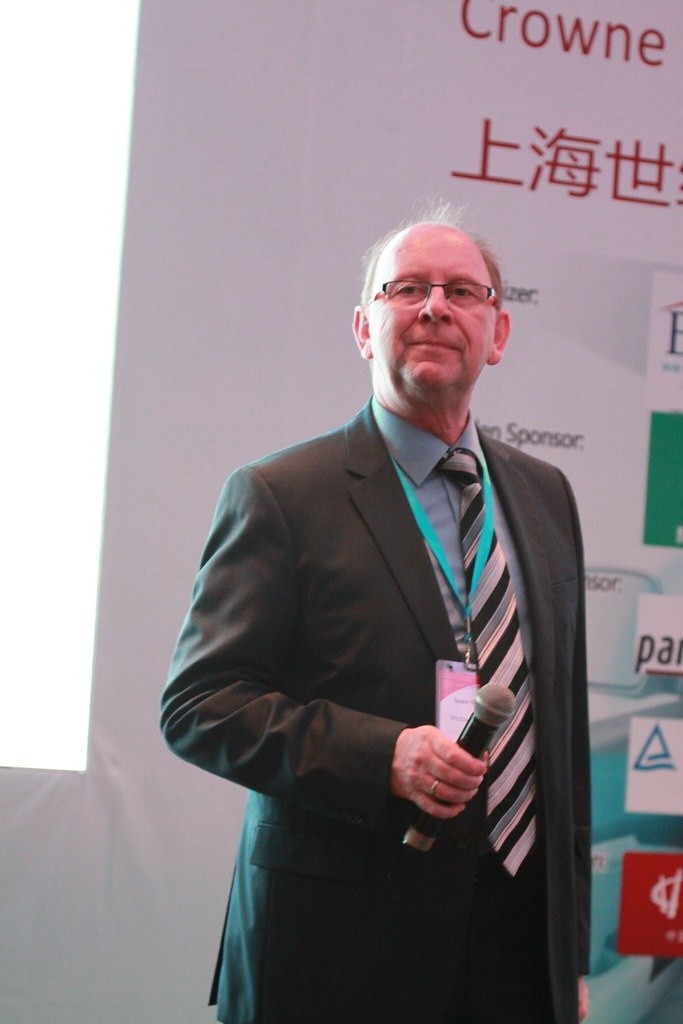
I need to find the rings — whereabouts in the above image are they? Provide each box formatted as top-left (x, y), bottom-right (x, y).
top-left (430, 779), bottom-right (439, 796)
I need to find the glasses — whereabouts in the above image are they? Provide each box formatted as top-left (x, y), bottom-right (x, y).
top-left (368, 279), bottom-right (497, 314)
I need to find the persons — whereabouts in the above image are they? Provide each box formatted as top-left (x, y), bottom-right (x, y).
top-left (157, 218), bottom-right (594, 1024)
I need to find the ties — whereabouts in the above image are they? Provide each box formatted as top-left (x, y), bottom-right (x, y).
top-left (433, 446), bottom-right (540, 876)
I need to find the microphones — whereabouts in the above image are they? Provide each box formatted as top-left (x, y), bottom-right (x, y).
top-left (402, 683), bottom-right (516, 852)
